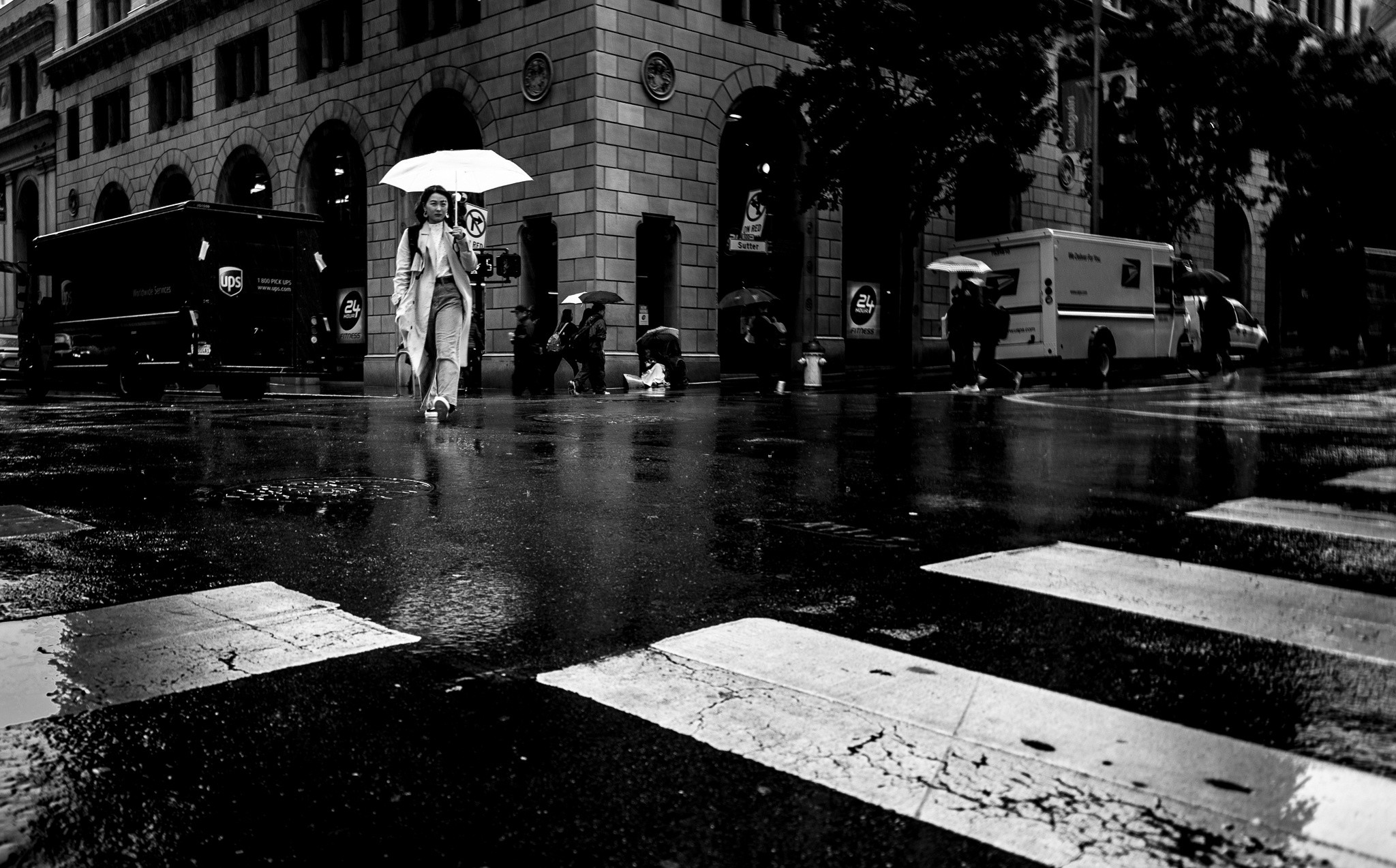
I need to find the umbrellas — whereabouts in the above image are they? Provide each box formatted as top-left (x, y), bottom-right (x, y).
top-left (379, 149), bottom-right (533, 226)
top-left (636, 326), bottom-right (680, 346)
top-left (559, 292), bottom-right (587, 305)
top-left (578, 291), bottom-right (624, 304)
top-left (926, 258), bottom-right (995, 275)
top-left (1172, 268), bottom-right (1230, 299)
top-left (716, 287), bottom-right (780, 309)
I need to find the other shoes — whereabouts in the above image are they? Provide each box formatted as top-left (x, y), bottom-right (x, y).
top-left (568, 380), bottom-right (576, 395)
top-left (959, 384), bottom-right (980, 393)
top-left (1187, 367), bottom-right (1202, 381)
top-left (594, 390), bottom-right (611, 395)
top-left (425, 407), bottom-right (439, 418)
top-left (1223, 369), bottom-right (1239, 380)
top-left (433, 397), bottom-right (449, 421)
top-left (1014, 371), bottom-right (1023, 393)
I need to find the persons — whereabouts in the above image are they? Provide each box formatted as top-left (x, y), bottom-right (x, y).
top-left (554, 309), bottom-right (579, 378)
top-left (405, 354), bottom-right (417, 396)
top-left (510, 304), bottom-right (545, 397)
top-left (947, 272), bottom-right (1023, 394)
top-left (462, 324), bottom-right (485, 396)
top-left (391, 185), bottom-right (479, 417)
top-left (1199, 292), bottom-right (1236, 375)
top-left (567, 304), bottom-right (610, 395)
top-left (648, 60), bottom-right (671, 92)
top-left (644, 340), bottom-right (689, 391)
top-left (740, 303), bottom-right (793, 395)
top-left (526, 61), bottom-right (546, 89)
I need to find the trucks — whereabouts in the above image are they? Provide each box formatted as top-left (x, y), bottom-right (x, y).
top-left (940, 228), bottom-right (1196, 385)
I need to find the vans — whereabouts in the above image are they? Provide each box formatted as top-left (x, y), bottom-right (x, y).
top-left (1181, 295), bottom-right (1270, 369)
top-left (0, 200), bottom-right (338, 405)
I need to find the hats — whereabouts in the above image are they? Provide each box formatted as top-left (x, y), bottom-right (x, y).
top-left (510, 305), bottom-right (527, 314)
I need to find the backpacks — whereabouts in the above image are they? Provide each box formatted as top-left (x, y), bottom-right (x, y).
top-left (991, 306), bottom-right (1010, 340)
top-left (762, 314), bottom-right (789, 350)
top-left (547, 322), bottom-right (570, 352)
top-left (569, 317), bottom-right (603, 362)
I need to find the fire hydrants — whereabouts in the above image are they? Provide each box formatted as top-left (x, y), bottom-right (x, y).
top-left (797, 337), bottom-right (827, 391)
